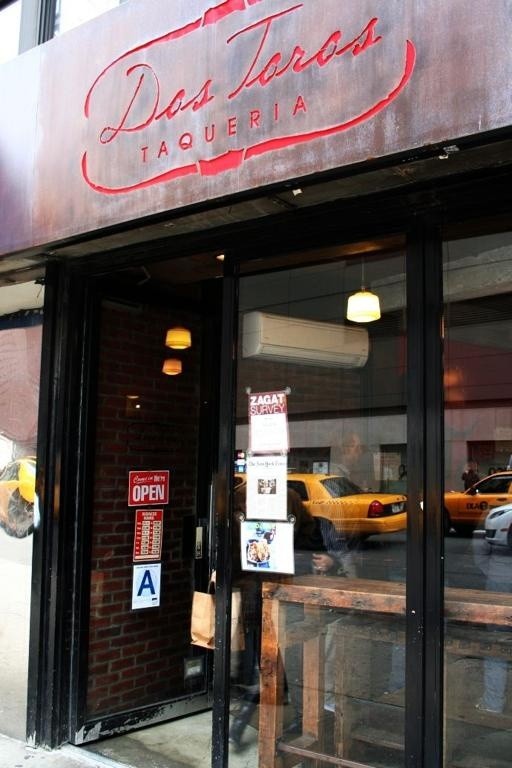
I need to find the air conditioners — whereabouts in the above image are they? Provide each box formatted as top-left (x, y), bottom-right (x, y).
top-left (243, 311), bottom-right (369, 370)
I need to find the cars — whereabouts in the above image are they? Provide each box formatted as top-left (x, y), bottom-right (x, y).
top-left (484, 502), bottom-right (512, 547)
top-left (233, 471), bottom-right (408, 550)
top-left (443, 470), bottom-right (512, 535)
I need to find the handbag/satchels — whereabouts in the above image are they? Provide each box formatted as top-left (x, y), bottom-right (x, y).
top-left (190, 581), bottom-right (245, 652)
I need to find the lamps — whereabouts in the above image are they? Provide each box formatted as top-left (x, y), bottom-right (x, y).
top-left (165, 325), bottom-right (191, 350)
top-left (162, 358), bottom-right (182, 376)
top-left (346, 263), bottom-right (381, 323)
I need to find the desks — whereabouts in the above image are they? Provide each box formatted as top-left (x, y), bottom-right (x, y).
top-left (258, 575), bottom-right (512, 768)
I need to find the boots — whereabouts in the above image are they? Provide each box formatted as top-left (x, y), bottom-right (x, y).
top-left (284, 681), bottom-right (303, 735)
top-left (228, 668), bottom-right (259, 753)
